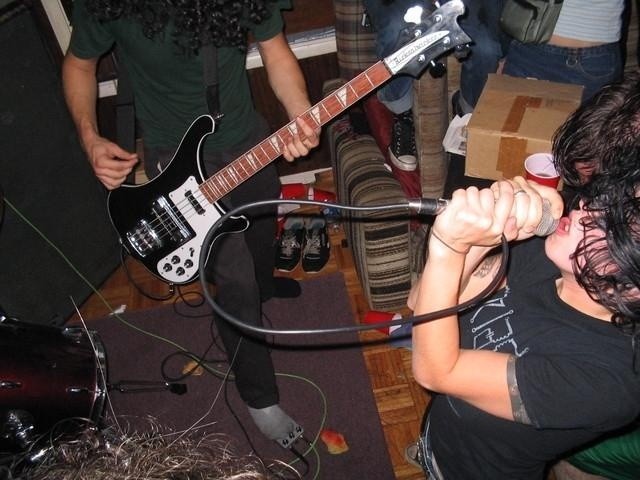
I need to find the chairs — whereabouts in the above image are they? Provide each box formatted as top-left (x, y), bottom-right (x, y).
top-left (323, 1), bottom-right (462, 312)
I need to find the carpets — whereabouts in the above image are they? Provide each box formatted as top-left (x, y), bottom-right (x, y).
top-left (67, 273), bottom-right (397, 479)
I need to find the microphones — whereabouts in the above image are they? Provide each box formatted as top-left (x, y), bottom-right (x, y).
top-left (408, 196), bottom-right (559, 237)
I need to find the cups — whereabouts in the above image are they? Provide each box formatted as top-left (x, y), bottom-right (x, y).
top-left (278, 183), bottom-right (306, 200)
top-left (523, 151), bottom-right (561, 188)
top-left (307, 186), bottom-right (336, 204)
top-left (362, 310), bottom-right (402, 335)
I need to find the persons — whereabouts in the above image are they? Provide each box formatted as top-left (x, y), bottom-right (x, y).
top-left (405, 77), bottom-right (640, 480)
top-left (502, 1), bottom-right (626, 105)
top-left (60, 1), bottom-right (322, 440)
top-left (563, 236), bottom-right (566, 241)
top-left (362, 0), bottom-right (501, 172)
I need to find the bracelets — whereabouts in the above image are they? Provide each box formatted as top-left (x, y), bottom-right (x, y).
top-left (429, 227), bottom-right (470, 256)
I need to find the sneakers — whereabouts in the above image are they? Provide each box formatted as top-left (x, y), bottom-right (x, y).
top-left (276, 217), bottom-right (306, 272)
top-left (452, 91), bottom-right (464, 119)
top-left (302, 219), bottom-right (330, 274)
top-left (388, 108), bottom-right (418, 171)
top-left (406, 445), bottom-right (422, 468)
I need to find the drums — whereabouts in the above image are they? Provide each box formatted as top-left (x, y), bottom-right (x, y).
top-left (1, 314), bottom-right (110, 478)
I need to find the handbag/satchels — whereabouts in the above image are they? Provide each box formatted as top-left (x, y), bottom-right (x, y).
top-left (501, 0), bottom-right (563, 45)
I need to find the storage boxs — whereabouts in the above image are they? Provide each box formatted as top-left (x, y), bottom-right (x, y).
top-left (464, 73), bottom-right (585, 193)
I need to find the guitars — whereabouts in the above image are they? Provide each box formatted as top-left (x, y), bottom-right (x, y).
top-left (105, 0), bottom-right (473, 280)
